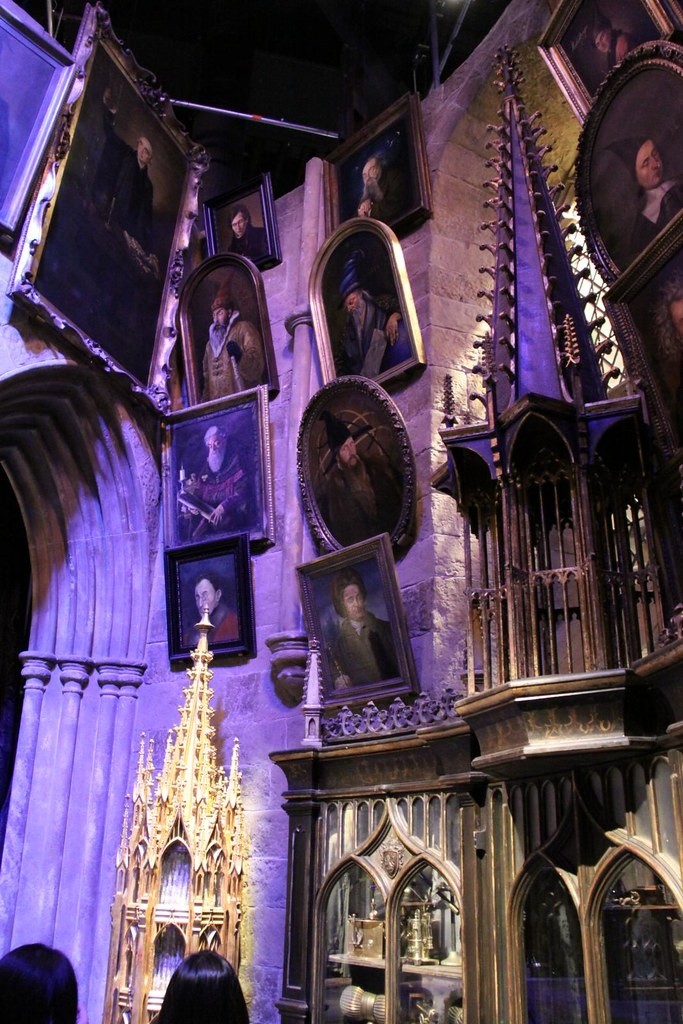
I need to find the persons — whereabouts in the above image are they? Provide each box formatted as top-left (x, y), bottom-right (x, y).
top-left (155, 950), bottom-right (249, 1024)
top-left (0, 943), bottom-right (78, 1024)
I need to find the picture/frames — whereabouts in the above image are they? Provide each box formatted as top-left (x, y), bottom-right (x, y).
top-left (322, 89), bottom-right (435, 239)
top-left (5, 0), bottom-right (211, 413)
top-left (163, 531), bottom-right (257, 664)
top-left (297, 375), bottom-right (417, 553)
top-left (294, 531), bottom-right (421, 711)
top-left (601, 207), bottom-right (683, 473)
top-left (574, 39), bottom-right (683, 287)
top-left (176, 251), bottom-right (280, 407)
top-left (308, 217), bottom-right (428, 384)
top-left (161, 383), bottom-right (276, 550)
top-left (0, 0), bottom-right (79, 233)
top-left (534, 0), bottom-right (683, 128)
top-left (202, 171), bottom-right (282, 266)
